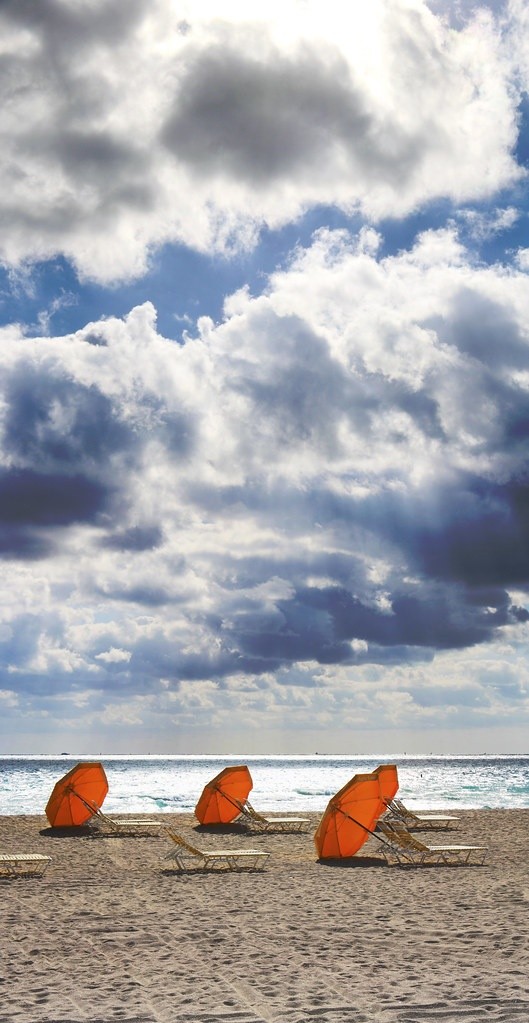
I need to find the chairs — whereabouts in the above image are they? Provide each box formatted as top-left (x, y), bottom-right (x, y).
top-left (0, 854), bottom-right (52, 878)
top-left (80, 800), bottom-right (167, 838)
top-left (382, 795), bottom-right (462, 833)
top-left (163, 827), bottom-right (271, 873)
top-left (374, 818), bottom-right (489, 872)
top-left (232, 797), bottom-right (312, 836)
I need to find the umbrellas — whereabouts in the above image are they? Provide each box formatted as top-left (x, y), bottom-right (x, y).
top-left (315, 774), bottom-right (413, 862)
top-left (45, 763), bottom-right (116, 831)
top-left (372, 765), bottom-right (407, 825)
top-left (195, 766), bottom-right (264, 830)
